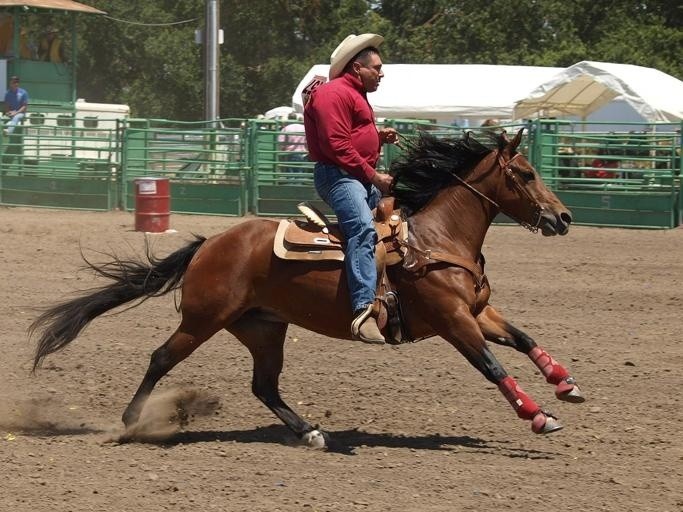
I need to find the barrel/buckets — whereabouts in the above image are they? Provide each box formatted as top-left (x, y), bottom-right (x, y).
top-left (132, 176), bottom-right (171, 233)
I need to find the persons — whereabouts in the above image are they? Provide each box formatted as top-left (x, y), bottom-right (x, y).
top-left (1, 75), bottom-right (27, 137)
top-left (215, 111), bottom-right (682, 186)
top-left (303, 33), bottom-right (399, 345)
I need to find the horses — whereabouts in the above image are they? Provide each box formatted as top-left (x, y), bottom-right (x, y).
top-left (24, 125), bottom-right (589, 453)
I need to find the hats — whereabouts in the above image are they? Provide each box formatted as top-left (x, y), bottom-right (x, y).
top-left (329, 34), bottom-right (384, 80)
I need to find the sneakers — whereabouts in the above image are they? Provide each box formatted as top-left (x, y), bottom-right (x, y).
top-left (358, 316), bottom-right (386, 344)
